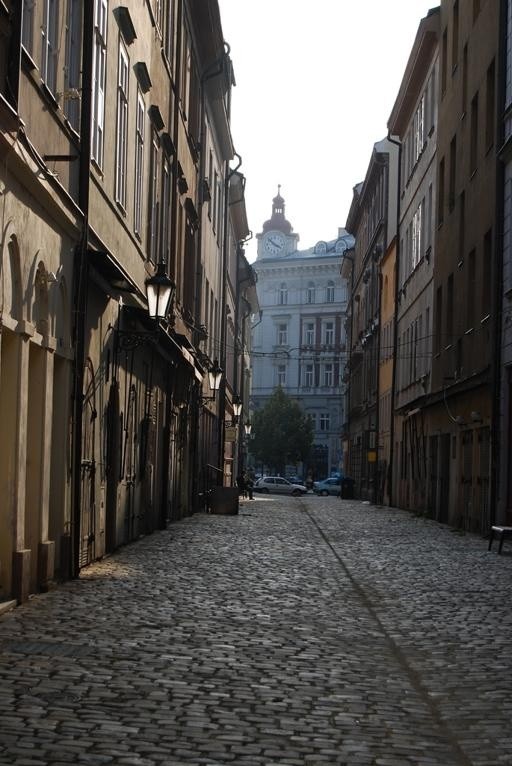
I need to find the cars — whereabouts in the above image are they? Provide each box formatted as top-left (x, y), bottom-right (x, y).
top-left (253, 473), bottom-right (341, 497)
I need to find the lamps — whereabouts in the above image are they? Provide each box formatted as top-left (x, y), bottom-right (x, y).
top-left (144, 261), bottom-right (254, 438)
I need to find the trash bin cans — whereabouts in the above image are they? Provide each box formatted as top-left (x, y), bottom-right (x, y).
top-left (341, 477), bottom-right (355, 499)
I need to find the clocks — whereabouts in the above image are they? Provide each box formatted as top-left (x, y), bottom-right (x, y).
top-left (264, 234), bottom-right (285, 254)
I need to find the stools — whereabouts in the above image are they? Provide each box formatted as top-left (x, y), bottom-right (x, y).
top-left (488, 526), bottom-right (511, 556)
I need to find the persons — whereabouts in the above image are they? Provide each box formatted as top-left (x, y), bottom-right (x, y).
top-left (237, 470), bottom-right (255, 501)
top-left (247, 466), bottom-right (255, 483)
top-left (304, 468), bottom-right (313, 490)
